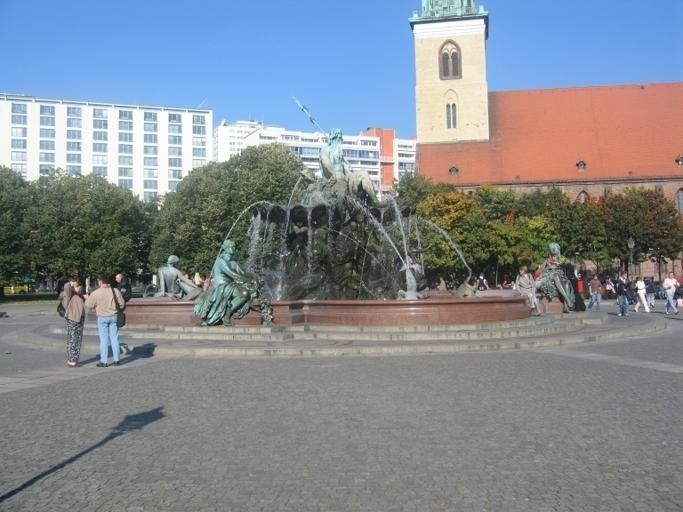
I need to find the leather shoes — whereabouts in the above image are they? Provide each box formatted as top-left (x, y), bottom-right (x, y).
top-left (97, 361), bottom-right (119, 367)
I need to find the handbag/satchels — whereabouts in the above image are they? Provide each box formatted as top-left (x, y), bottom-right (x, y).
top-left (117, 310), bottom-right (125, 327)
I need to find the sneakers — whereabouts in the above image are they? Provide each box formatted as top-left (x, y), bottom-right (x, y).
top-left (666, 309), bottom-right (679, 315)
top-left (618, 313), bottom-right (629, 317)
top-left (634, 303), bottom-right (654, 312)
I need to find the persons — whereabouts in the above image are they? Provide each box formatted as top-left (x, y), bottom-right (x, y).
top-left (60, 280), bottom-right (86, 368)
top-left (436, 252), bottom-right (680, 318)
top-left (541, 241), bottom-right (583, 314)
top-left (155, 239), bottom-right (274, 326)
top-left (317, 126), bottom-right (387, 208)
top-left (85, 272), bottom-right (132, 368)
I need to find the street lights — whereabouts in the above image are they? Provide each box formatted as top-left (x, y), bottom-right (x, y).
top-left (627, 236), bottom-right (635, 283)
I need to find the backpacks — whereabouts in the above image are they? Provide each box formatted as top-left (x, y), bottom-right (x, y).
top-left (57, 294), bottom-right (85, 316)
